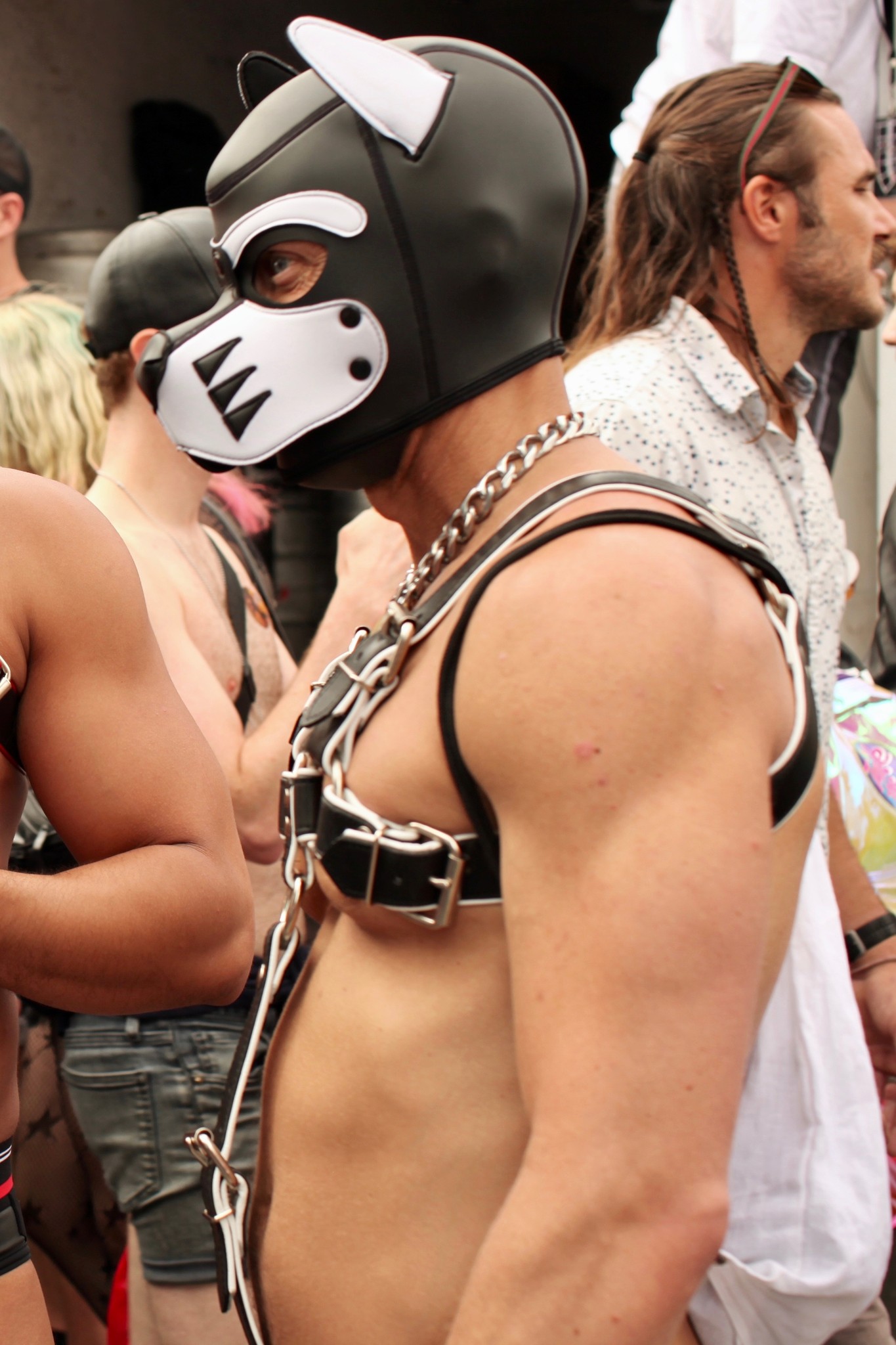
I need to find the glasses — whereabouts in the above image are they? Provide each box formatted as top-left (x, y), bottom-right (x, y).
top-left (738, 57), bottom-right (823, 212)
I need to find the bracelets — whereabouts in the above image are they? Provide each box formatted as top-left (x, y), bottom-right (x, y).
top-left (842, 914), bottom-right (896, 961)
top-left (851, 958), bottom-right (896, 978)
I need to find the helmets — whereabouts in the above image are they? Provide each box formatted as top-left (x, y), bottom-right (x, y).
top-left (84, 207), bottom-right (224, 359)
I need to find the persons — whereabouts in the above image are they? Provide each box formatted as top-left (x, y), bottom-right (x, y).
top-left (0, 134), bottom-right (422, 1345)
top-left (607, 0), bottom-right (895, 347)
top-left (139, 16), bottom-right (832, 1345)
top-left (563, 62), bottom-right (896, 1344)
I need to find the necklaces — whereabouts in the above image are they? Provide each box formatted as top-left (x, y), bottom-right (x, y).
top-left (395, 411), bottom-right (599, 602)
